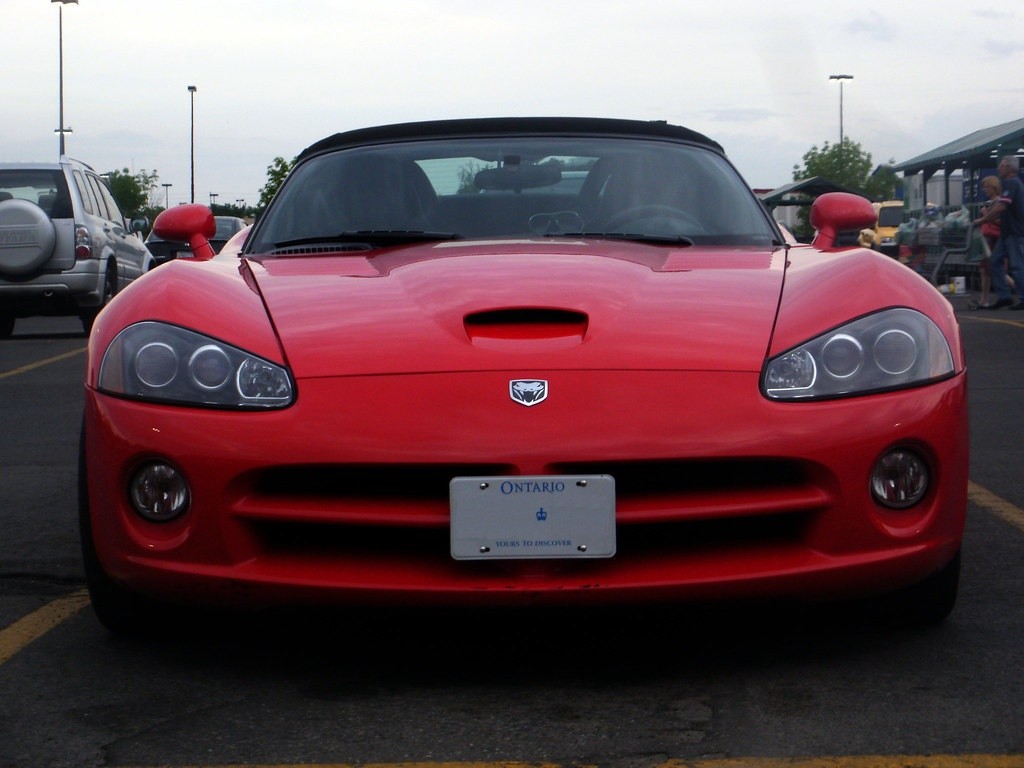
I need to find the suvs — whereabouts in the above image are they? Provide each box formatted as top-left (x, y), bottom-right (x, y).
top-left (856, 199), bottom-right (941, 255)
top-left (0, 154), bottom-right (160, 335)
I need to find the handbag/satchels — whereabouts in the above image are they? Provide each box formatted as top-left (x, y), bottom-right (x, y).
top-left (966, 227), bottom-right (992, 262)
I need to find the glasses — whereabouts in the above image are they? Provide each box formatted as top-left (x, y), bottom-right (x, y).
top-left (999, 164), bottom-right (1009, 168)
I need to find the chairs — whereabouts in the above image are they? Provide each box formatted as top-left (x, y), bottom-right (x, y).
top-left (318, 159), bottom-right (445, 233)
top-left (581, 167), bottom-right (691, 233)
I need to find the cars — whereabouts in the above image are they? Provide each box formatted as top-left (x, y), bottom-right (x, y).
top-left (141, 215), bottom-right (252, 264)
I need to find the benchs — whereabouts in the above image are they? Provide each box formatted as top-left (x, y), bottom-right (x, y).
top-left (438, 195), bottom-right (581, 238)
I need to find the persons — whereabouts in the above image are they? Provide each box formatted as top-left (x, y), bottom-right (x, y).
top-left (977, 176), bottom-right (1015, 306)
top-left (971, 154), bottom-right (1024, 310)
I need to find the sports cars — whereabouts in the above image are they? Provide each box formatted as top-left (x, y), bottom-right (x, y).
top-left (79, 115), bottom-right (970, 635)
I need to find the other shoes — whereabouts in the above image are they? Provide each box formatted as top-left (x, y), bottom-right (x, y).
top-left (968, 301), bottom-right (990, 309)
top-left (1009, 302), bottom-right (1024, 310)
top-left (988, 297), bottom-right (1014, 310)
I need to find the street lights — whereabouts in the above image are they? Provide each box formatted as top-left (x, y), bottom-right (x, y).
top-left (210, 193), bottom-right (219, 204)
top-left (187, 85), bottom-right (197, 205)
top-left (235, 199), bottom-right (244, 208)
top-left (829, 73), bottom-right (853, 156)
top-left (161, 184), bottom-right (173, 209)
top-left (50, 0), bottom-right (79, 161)
